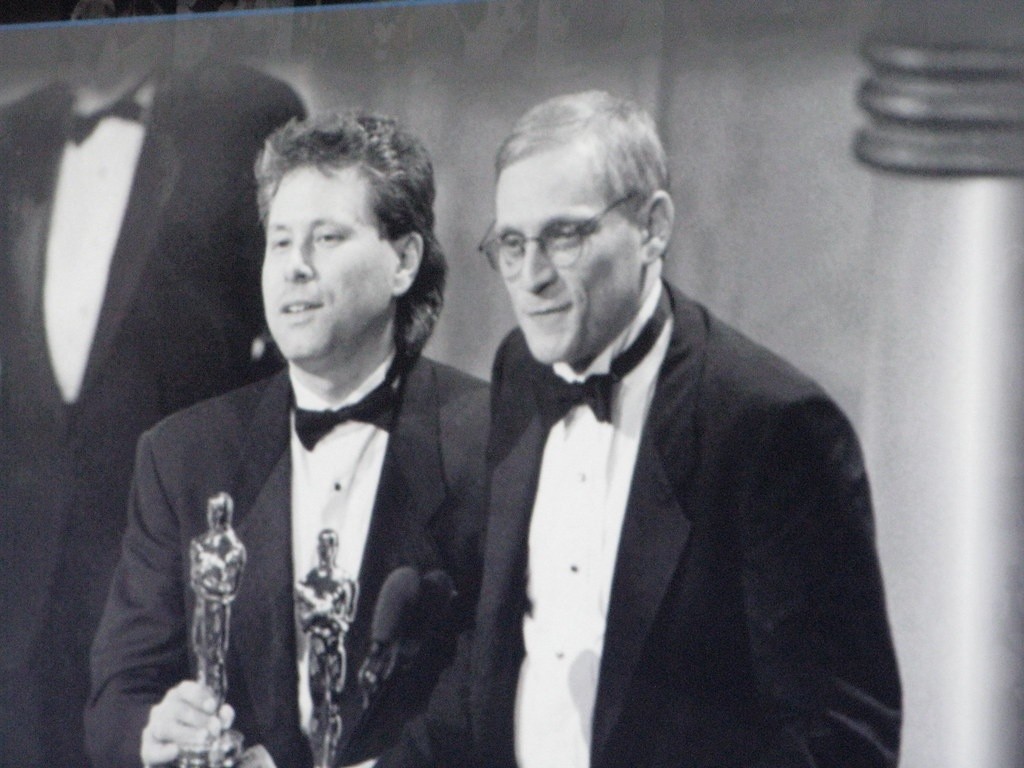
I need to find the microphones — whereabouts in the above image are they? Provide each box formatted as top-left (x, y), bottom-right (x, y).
top-left (332, 566), bottom-right (456, 768)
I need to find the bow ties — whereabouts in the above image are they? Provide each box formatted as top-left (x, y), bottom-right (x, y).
top-left (527, 283), bottom-right (671, 423)
top-left (289, 352), bottom-right (401, 451)
top-left (68, 68), bottom-right (155, 146)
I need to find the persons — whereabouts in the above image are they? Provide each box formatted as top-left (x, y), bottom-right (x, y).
top-left (298, 528), bottom-right (359, 768)
top-left (91, 102), bottom-right (490, 768)
top-left (0, 0), bottom-right (305, 768)
top-left (352, 90), bottom-right (905, 768)
top-left (176, 489), bottom-right (245, 768)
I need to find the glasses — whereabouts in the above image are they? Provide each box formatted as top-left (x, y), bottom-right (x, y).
top-left (477, 191), bottom-right (643, 280)
